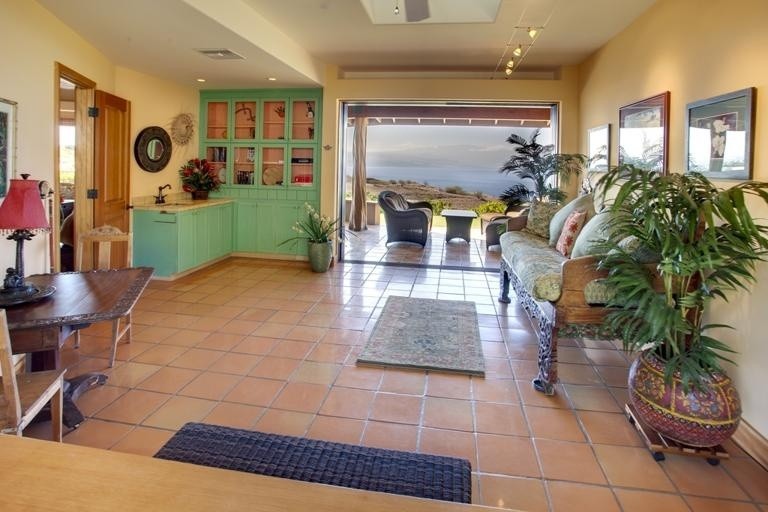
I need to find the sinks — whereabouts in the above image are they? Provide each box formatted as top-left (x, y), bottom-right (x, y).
top-left (157, 202), bottom-right (190, 208)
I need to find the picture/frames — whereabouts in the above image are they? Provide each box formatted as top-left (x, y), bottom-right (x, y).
top-left (683, 85), bottom-right (754, 183)
top-left (584, 122), bottom-right (610, 178)
top-left (0, 96), bottom-right (19, 202)
top-left (615, 90), bottom-right (668, 178)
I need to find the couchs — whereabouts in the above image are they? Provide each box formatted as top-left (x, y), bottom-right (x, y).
top-left (376, 190), bottom-right (432, 248)
top-left (479, 201), bottom-right (520, 235)
top-left (495, 192), bottom-right (708, 397)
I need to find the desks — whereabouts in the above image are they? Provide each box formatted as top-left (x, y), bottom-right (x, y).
top-left (0, 265), bottom-right (154, 433)
top-left (0, 434), bottom-right (520, 512)
top-left (440, 208), bottom-right (478, 246)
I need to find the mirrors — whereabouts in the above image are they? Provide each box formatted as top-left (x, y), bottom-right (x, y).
top-left (133, 125), bottom-right (172, 173)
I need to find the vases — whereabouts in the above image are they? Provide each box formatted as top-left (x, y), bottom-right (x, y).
top-left (308, 241), bottom-right (332, 275)
top-left (188, 190), bottom-right (209, 201)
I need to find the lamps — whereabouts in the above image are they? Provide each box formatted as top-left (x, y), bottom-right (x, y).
top-left (502, 69), bottom-right (514, 75)
top-left (506, 64), bottom-right (515, 69)
top-left (0, 179), bottom-right (52, 305)
top-left (512, 46), bottom-right (524, 58)
top-left (526, 26), bottom-right (538, 41)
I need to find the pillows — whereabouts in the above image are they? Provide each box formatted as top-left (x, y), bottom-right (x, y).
top-left (522, 196), bottom-right (558, 240)
top-left (570, 206), bottom-right (638, 260)
top-left (546, 194), bottom-right (595, 250)
top-left (603, 234), bottom-right (642, 257)
top-left (554, 211), bottom-right (587, 258)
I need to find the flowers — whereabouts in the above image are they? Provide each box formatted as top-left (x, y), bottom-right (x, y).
top-left (179, 157), bottom-right (221, 192)
top-left (277, 200), bottom-right (357, 251)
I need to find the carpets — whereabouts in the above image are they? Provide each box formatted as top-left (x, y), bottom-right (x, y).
top-left (151, 421), bottom-right (472, 504)
top-left (354, 292), bottom-right (487, 377)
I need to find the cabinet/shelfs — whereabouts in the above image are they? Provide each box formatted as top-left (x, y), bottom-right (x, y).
top-left (198, 86), bottom-right (319, 263)
top-left (132, 196), bottom-right (234, 282)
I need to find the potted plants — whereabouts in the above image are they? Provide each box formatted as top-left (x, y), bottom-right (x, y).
top-left (585, 163), bottom-right (768, 452)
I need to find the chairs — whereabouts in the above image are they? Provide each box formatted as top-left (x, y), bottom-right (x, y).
top-left (0, 309), bottom-right (70, 444)
top-left (72, 224), bottom-right (135, 369)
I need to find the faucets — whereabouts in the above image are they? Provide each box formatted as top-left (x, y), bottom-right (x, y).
top-left (158, 184), bottom-right (171, 204)
